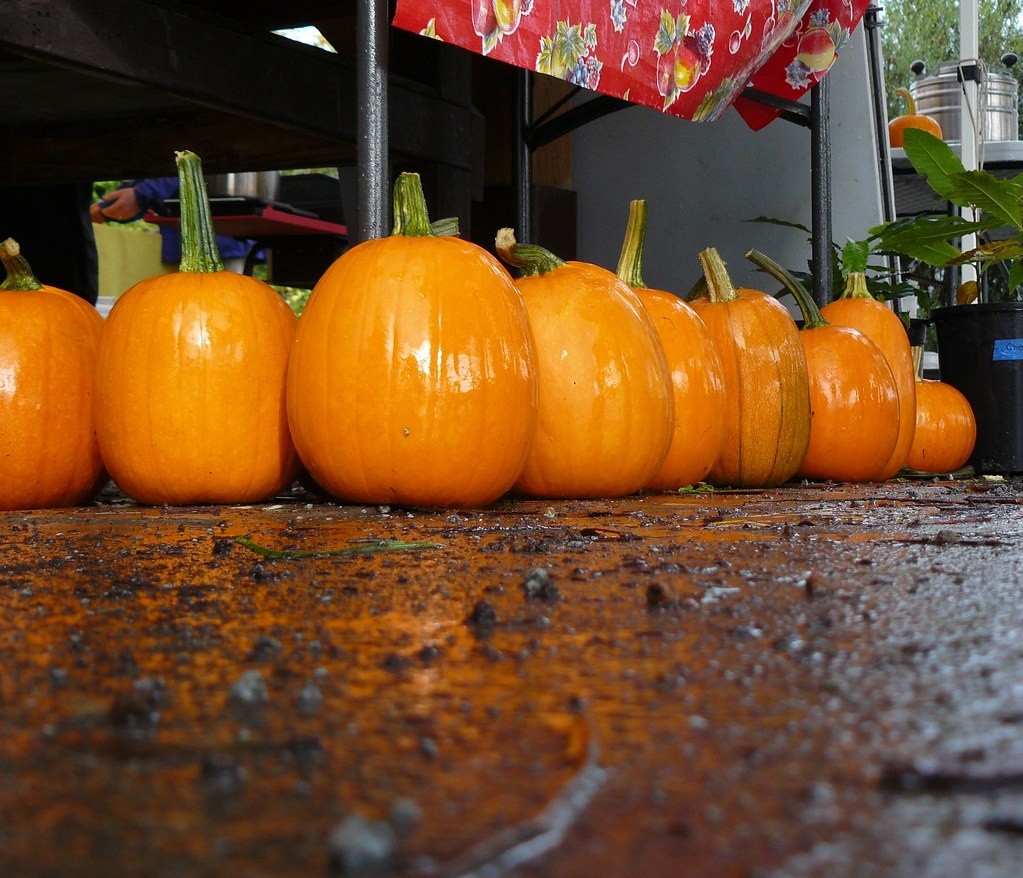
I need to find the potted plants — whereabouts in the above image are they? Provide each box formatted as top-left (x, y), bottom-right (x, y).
top-left (880, 126), bottom-right (1023, 476)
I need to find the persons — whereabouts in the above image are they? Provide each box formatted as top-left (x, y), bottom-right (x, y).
top-left (90, 176), bottom-right (266, 275)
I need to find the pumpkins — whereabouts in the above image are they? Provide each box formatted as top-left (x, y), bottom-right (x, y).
top-left (888, 87), bottom-right (943, 147)
top-left (0, 150), bottom-right (976, 510)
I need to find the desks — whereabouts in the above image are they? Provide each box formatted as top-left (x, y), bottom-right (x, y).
top-left (0, 0), bottom-right (486, 185)
top-left (146, 205), bottom-right (350, 275)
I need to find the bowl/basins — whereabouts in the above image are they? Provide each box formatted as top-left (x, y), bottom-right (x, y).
top-left (206, 171), bottom-right (281, 199)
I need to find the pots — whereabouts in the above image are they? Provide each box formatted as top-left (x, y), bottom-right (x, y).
top-left (909, 52), bottom-right (1019, 142)
